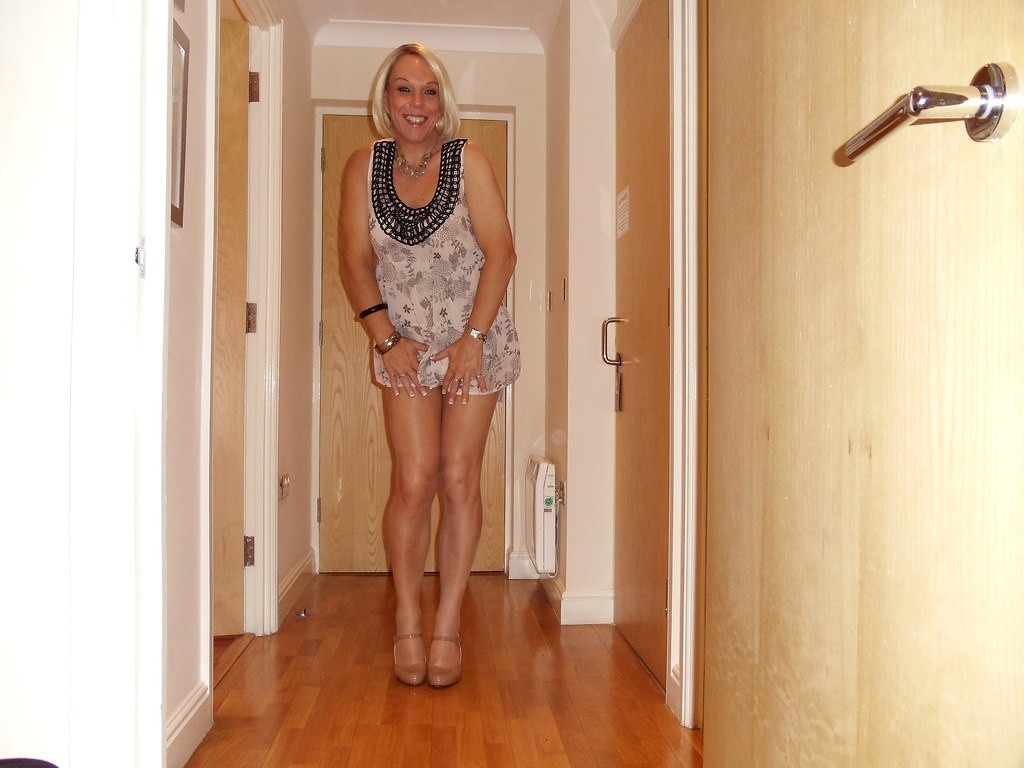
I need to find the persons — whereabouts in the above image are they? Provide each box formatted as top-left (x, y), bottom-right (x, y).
top-left (337, 44), bottom-right (522, 688)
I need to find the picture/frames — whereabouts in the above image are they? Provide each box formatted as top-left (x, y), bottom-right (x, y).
top-left (170, 17), bottom-right (190, 230)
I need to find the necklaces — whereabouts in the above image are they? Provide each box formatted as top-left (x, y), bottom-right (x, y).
top-left (394, 140), bottom-right (438, 179)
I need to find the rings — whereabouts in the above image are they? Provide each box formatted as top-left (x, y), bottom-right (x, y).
top-left (399, 373), bottom-right (407, 378)
top-left (475, 374), bottom-right (482, 378)
top-left (453, 378), bottom-right (460, 382)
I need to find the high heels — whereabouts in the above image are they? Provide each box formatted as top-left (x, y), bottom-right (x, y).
top-left (427, 632), bottom-right (463, 686)
top-left (393, 632), bottom-right (428, 684)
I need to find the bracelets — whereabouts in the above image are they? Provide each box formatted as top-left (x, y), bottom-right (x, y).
top-left (464, 325), bottom-right (486, 342)
top-left (376, 331), bottom-right (401, 354)
top-left (359, 303), bottom-right (388, 319)
